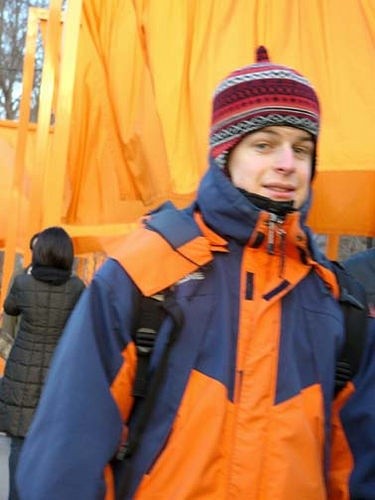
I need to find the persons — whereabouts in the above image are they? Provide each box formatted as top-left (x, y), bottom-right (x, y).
top-left (0, 231), bottom-right (79, 362)
top-left (12, 45), bottom-right (375, 499)
top-left (342, 245), bottom-right (375, 317)
top-left (0, 225), bottom-right (86, 499)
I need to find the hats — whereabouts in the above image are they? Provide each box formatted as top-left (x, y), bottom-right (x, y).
top-left (211, 44), bottom-right (320, 195)
